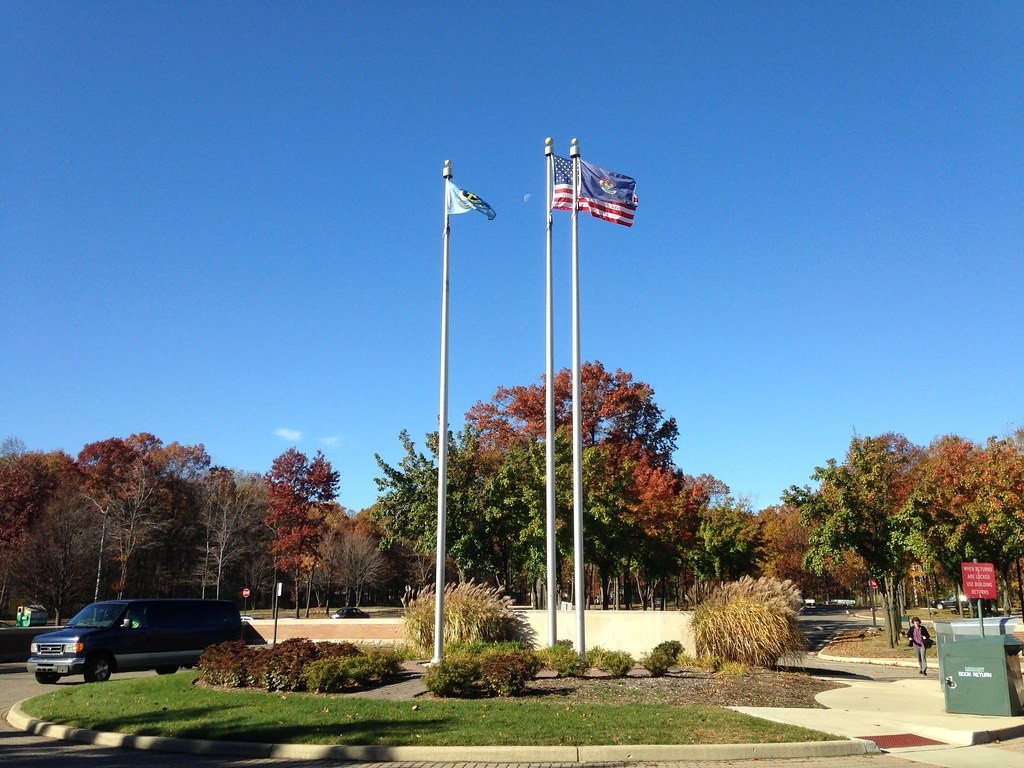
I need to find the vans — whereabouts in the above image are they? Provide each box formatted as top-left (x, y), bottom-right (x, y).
top-left (26, 598), bottom-right (244, 684)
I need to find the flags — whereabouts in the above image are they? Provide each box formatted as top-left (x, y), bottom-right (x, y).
top-left (551, 154), bottom-right (579, 210)
top-left (447, 179), bottom-right (497, 220)
top-left (577, 159), bottom-right (639, 228)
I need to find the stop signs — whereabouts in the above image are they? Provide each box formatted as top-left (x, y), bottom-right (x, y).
top-left (869, 576), bottom-right (879, 590)
top-left (243, 588), bottom-right (251, 598)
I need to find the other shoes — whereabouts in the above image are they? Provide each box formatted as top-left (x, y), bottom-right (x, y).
top-left (919, 671), bottom-right (927, 675)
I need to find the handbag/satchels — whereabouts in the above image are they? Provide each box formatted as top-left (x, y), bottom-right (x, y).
top-left (923, 639), bottom-right (934, 649)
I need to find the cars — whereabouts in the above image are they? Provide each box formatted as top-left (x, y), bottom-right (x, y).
top-left (930, 593), bottom-right (969, 610)
top-left (330, 606), bottom-right (370, 619)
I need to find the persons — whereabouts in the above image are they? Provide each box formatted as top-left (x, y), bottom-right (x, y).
top-left (907, 616), bottom-right (930, 676)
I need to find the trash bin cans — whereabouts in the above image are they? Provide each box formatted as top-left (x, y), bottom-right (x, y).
top-left (938, 634), bottom-right (1024, 717)
top-left (16, 605), bottom-right (48, 627)
